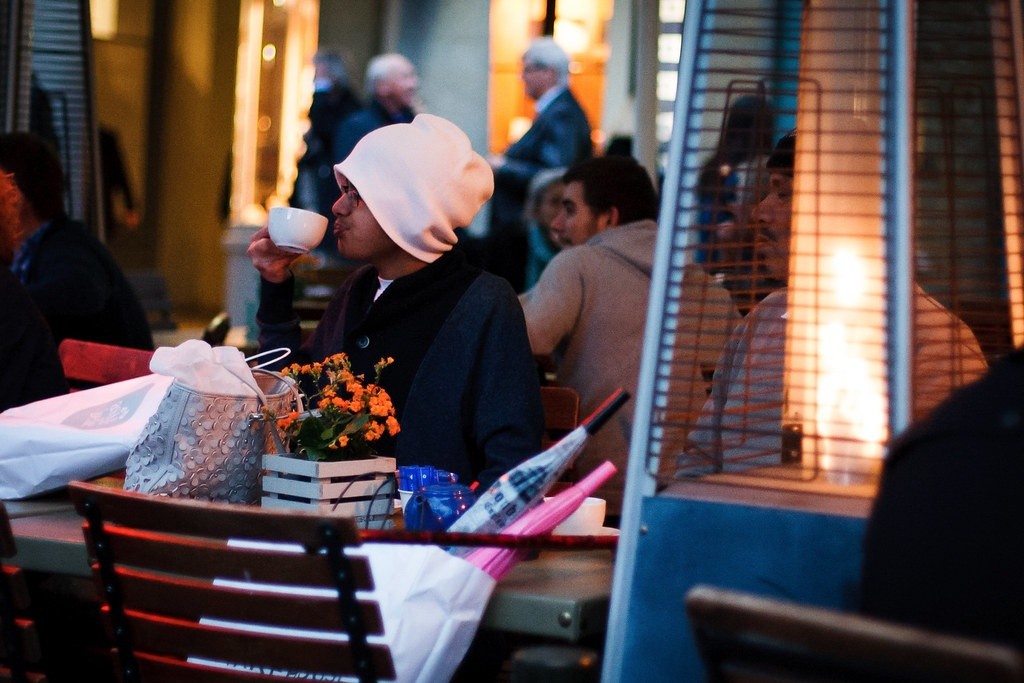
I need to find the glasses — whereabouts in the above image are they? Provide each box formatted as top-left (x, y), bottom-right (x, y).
top-left (341, 180), bottom-right (361, 208)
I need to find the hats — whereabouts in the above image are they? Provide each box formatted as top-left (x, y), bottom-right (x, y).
top-left (333, 113), bottom-right (494, 263)
top-left (766, 126), bottom-right (794, 174)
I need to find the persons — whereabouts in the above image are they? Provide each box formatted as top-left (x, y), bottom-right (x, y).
top-left (247, 112), bottom-right (542, 498)
top-left (514, 153), bottom-right (743, 531)
top-left (0, 130), bottom-right (155, 414)
top-left (290, 33), bottom-right (778, 316)
top-left (861, 343), bottom-right (1024, 650)
top-left (668, 123), bottom-right (990, 484)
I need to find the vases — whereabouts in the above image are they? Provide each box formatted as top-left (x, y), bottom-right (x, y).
top-left (259, 452), bottom-right (400, 527)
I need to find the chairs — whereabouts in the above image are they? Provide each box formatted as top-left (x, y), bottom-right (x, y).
top-left (70, 478), bottom-right (401, 683)
top-left (537, 380), bottom-right (581, 499)
top-left (61, 340), bottom-right (160, 405)
top-left (0, 500), bottom-right (50, 682)
top-left (683, 585), bottom-right (1024, 683)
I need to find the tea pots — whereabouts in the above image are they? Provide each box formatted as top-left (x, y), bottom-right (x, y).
top-left (403, 472), bottom-right (475, 531)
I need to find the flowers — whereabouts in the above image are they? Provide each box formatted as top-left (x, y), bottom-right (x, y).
top-left (267, 352), bottom-right (406, 454)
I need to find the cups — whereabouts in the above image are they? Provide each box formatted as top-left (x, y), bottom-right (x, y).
top-left (544, 497), bottom-right (607, 536)
top-left (268, 207), bottom-right (328, 254)
top-left (399, 487), bottom-right (415, 512)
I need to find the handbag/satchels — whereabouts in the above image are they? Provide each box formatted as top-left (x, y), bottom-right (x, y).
top-left (0, 346), bottom-right (290, 501)
top-left (121, 366), bottom-right (303, 506)
top-left (186, 471), bottom-right (498, 683)
